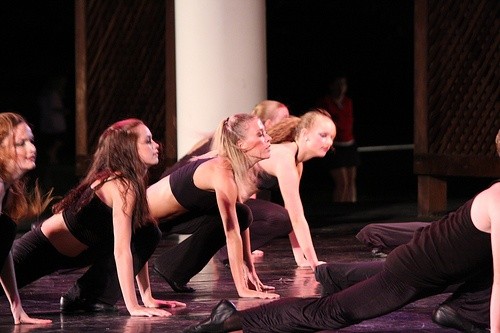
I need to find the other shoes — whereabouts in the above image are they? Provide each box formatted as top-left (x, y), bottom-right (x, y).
top-left (152, 263), bottom-right (195, 293)
top-left (314, 266), bottom-right (329, 298)
top-left (370, 245), bottom-right (388, 259)
top-left (432, 303), bottom-right (490, 333)
top-left (182, 298), bottom-right (236, 332)
top-left (59, 296), bottom-right (117, 314)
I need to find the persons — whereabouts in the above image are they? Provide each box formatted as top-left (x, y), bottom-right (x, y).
top-left (159, 101), bottom-right (291, 178)
top-left (357, 221), bottom-right (437, 257)
top-left (314, 262), bottom-right (385, 299)
top-left (0, 117), bottom-right (186, 318)
top-left (218, 109), bottom-right (336, 272)
top-left (0, 111), bottom-right (52, 325)
top-left (187, 129), bottom-right (500, 333)
top-left (143, 113), bottom-right (281, 300)
top-left (320, 74), bottom-right (360, 205)
